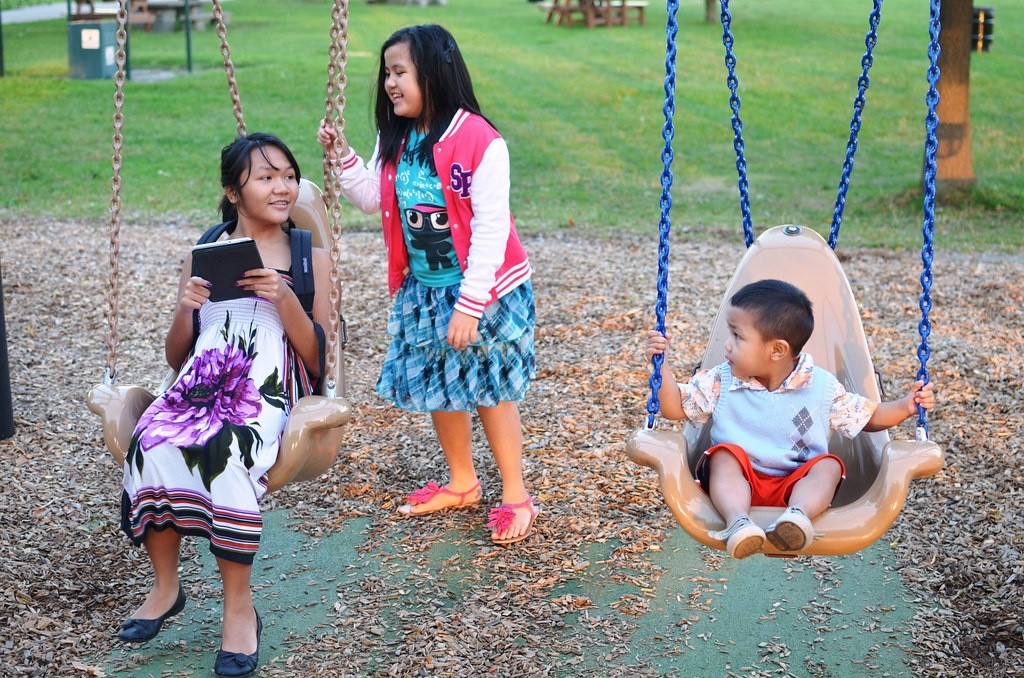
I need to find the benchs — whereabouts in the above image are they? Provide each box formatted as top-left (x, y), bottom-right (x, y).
top-left (70, 0), bottom-right (156, 34)
top-left (173, 12), bottom-right (231, 32)
top-left (536, 0), bottom-right (650, 30)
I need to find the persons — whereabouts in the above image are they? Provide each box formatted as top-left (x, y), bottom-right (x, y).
top-left (644, 277), bottom-right (936, 561)
top-left (316, 23), bottom-right (539, 545)
top-left (117, 132), bottom-right (332, 678)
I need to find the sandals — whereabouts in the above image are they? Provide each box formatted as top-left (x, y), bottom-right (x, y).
top-left (398, 482), bottom-right (482, 517)
top-left (487, 498), bottom-right (539, 543)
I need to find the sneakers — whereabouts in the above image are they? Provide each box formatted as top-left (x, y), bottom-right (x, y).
top-left (765, 507), bottom-right (815, 552)
top-left (709, 515), bottom-right (765, 561)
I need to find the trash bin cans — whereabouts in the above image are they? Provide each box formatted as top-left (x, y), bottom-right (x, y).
top-left (67, 0), bottom-right (124, 79)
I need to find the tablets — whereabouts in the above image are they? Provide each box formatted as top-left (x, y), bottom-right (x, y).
top-left (191, 237), bottom-right (267, 302)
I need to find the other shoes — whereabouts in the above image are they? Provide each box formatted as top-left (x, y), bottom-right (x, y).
top-left (117, 580), bottom-right (186, 643)
top-left (214, 608), bottom-right (262, 678)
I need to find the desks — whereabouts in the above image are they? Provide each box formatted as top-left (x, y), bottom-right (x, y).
top-left (126, 0), bottom-right (212, 35)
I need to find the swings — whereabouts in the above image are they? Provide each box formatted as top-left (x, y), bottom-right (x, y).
top-left (622, 0), bottom-right (953, 559)
top-left (83, 0), bottom-right (352, 496)
